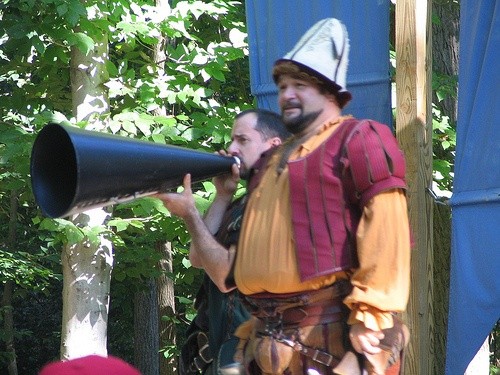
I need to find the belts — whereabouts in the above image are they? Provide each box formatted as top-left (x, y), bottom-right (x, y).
top-left (254, 306), bottom-right (347, 330)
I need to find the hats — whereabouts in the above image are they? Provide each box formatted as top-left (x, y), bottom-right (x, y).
top-left (271, 13), bottom-right (351, 110)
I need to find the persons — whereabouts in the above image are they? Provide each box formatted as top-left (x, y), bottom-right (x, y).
top-left (149, 107), bottom-right (292, 375)
top-left (221, 17), bottom-right (415, 375)
